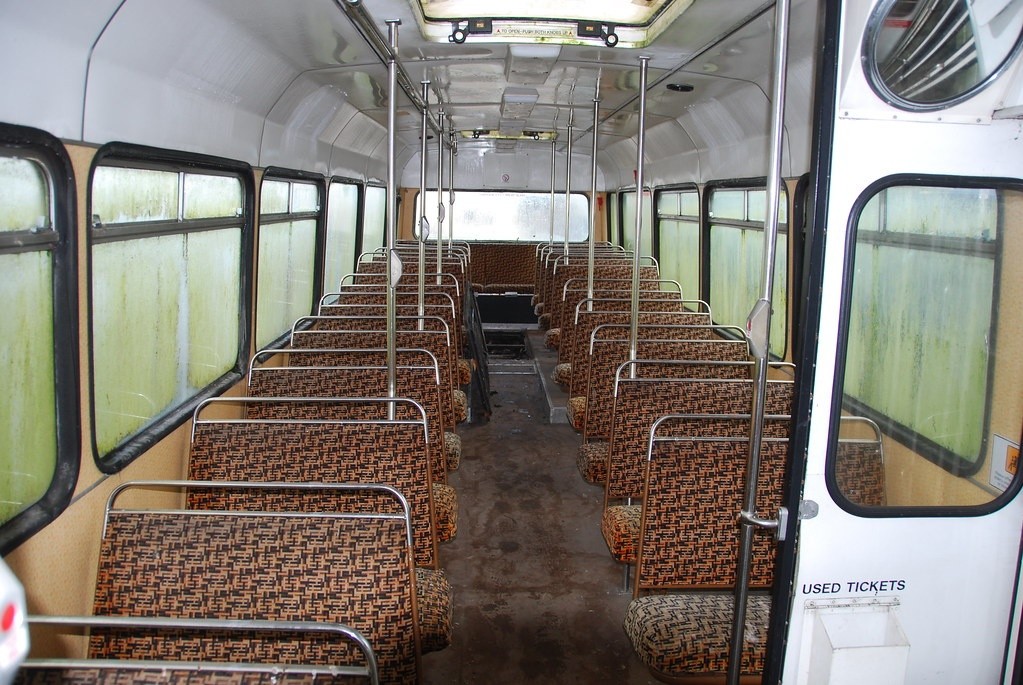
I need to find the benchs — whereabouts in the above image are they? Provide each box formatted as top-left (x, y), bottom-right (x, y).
top-left (20, 238), bottom-right (886, 685)
